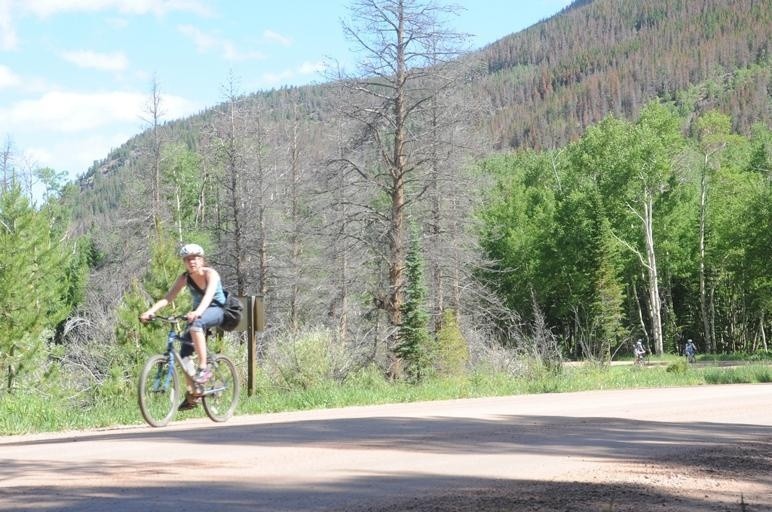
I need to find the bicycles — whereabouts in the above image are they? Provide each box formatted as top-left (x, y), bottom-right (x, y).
top-left (633, 351), bottom-right (652, 368)
top-left (686, 351), bottom-right (696, 364)
top-left (136, 313), bottom-right (243, 429)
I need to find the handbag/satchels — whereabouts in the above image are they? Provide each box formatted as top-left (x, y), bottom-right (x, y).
top-left (219, 289), bottom-right (244, 332)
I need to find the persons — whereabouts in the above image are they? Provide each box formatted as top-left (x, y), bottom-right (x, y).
top-left (633, 338), bottom-right (646, 350)
top-left (685, 339), bottom-right (696, 360)
top-left (633, 342), bottom-right (645, 361)
top-left (138, 242), bottom-right (226, 411)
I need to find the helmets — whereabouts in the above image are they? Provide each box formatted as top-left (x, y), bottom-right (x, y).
top-left (179, 244), bottom-right (204, 259)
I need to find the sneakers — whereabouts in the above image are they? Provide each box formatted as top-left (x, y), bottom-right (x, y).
top-left (177, 398), bottom-right (198, 411)
top-left (192, 367), bottom-right (212, 383)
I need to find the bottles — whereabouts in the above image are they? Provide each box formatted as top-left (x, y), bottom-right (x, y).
top-left (183, 356), bottom-right (197, 376)
